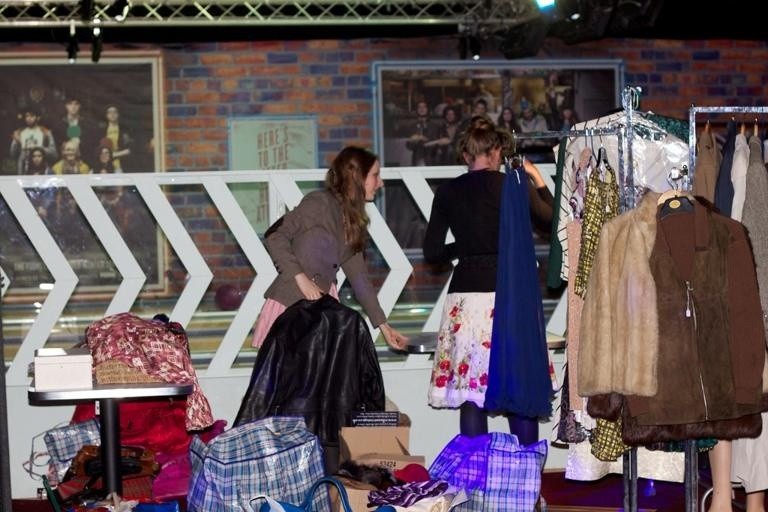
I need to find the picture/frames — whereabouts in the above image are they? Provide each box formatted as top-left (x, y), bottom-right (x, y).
top-left (1, 51), bottom-right (172, 304)
top-left (226, 111), bottom-right (319, 246)
top-left (372, 57), bottom-right (627, 264)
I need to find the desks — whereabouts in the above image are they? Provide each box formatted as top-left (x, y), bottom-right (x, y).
top-left (27, 383), bottom-right (194, 504)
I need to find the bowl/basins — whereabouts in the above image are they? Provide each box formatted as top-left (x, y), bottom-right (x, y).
top-left (215, 285), bottom-right (241, 310)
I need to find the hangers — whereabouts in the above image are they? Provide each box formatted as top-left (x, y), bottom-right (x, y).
top-left (619, 85), bottom-right (645, 123)
top-left (656, 166), bottom-right (705, 208)
top-left (566, 114), bottom-right (619, 165)
top-left (696, 106), bottom-right (768, 166)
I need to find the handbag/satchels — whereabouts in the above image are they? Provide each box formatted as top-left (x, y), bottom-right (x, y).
top-left (428, 432), bottom-right (547, 512)
top-left (249, 477), bottom-right (397, 512)
top-left (45, 415), bottom-right (104, 482)
top-left (188, 419), bottom-right (332, 512)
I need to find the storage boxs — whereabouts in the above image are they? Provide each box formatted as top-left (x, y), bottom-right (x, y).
top-left (29, 353), bottom-right (95, 392)
top-left (331, 393), bottom-right (426, 512)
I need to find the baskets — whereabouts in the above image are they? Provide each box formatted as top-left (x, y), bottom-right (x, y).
top-left (96, 359), bottom-right (162, 386)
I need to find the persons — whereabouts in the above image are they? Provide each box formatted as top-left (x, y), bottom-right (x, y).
top-left (383, 90), bottom-right (579, 165)
top-left (426, 115), bottom-right (556, 512)
top-left (251, 145), bottom-right (409, 351)
top-left (0, 95), bottom-right (138, 250)
